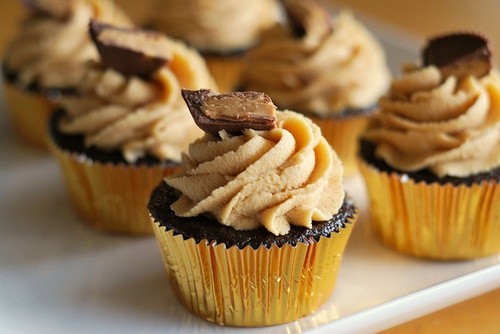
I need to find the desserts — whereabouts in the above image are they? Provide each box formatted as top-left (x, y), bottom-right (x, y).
top-left (140, 0), bottom-right (293, 93)
top-left (44, 18), bottom-right (221, 239)
top-left (231, 0), bottom-right (396, 180)
top-left (148, 88), bottom-right (361, 329)
top-left (0, 0), bottom-right (136, 149)
top-left (356, 31), bottom-right (500, 262)
